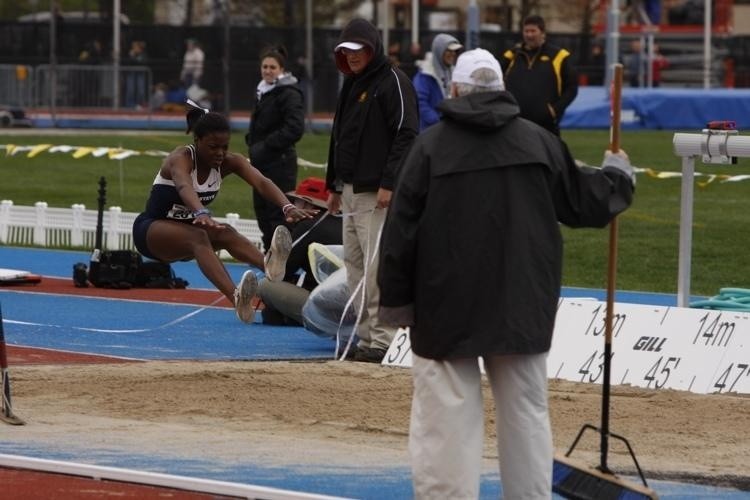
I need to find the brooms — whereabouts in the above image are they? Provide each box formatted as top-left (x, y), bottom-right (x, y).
top-left (553, 62), bottom-right (658, 500)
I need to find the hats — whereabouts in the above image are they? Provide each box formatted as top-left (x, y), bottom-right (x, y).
top-left (288, 178), bottom-right (328, 208)
top-left (449, 43), bottom-right (463, 52)
top-left (451, 48), bottom-right (503, 87)
top-left (335, 41), bottom-right (364, 53)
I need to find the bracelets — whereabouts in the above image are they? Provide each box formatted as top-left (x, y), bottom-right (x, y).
top-left (195, 208), bottom-right (213, 218)
top-left (282, 204), bottom-right (296, 217)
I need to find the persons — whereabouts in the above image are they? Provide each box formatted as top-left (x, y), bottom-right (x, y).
top-left (133, 112), bottom-right (320, 325)
top-left (324, 17), bottom-right (420, 364)
top-left (375, 44), bottom-right (637, 499)
top-left (580, 38), bottom-right (671, 90)
top-left (1, 30), bottom-right (217, 116)
top-left (411, 31), bottom-right (464, 133)
top-left (244, 42), bottom-right (305, 245)
top-left (505, 14), bottom-right (579, 137)
top-left (261, 177), bottom-right (344, 326)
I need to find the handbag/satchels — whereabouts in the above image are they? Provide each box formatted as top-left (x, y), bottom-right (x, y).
top-left (90, 252), bottom-right (144, 288)
top-left (142, 260), bottom-right (175, 289)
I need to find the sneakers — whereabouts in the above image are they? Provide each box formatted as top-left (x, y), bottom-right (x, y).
top-left (263, 226), bottom-right (292, 282)
top-left (234, 270), bottom-right (258, 323)
top-left (338, 344), bottom-right (385, 362)
